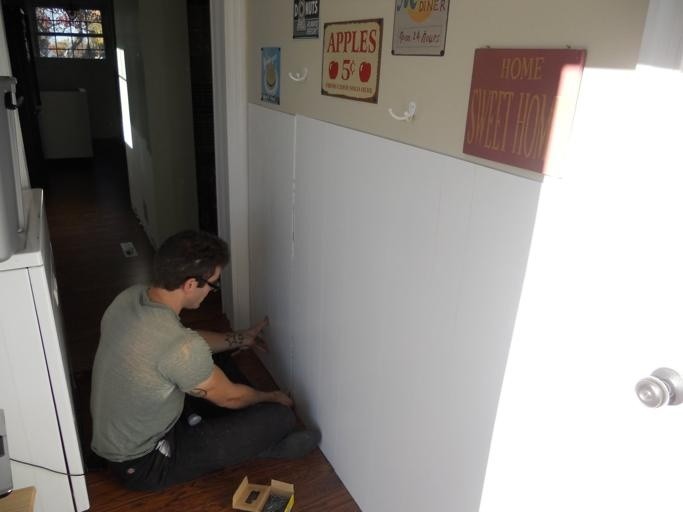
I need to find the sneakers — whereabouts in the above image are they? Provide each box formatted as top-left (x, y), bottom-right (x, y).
top-left (274, 431), bottom-right (315, 461)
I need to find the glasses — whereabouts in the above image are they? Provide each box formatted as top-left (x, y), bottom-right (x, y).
top-left (187, 276), bottom-right (221, 293)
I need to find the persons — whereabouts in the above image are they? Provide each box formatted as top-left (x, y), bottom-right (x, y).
top-left (90, 230), bottom-right (321, 490)
